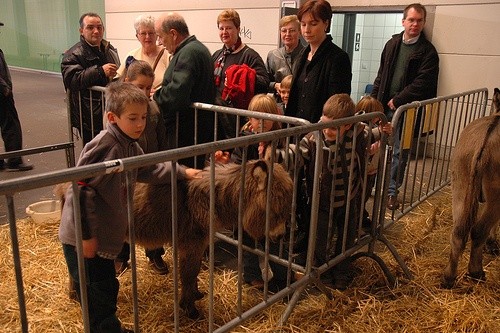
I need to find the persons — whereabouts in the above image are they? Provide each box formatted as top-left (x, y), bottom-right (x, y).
top-left (214, 94), bottom-right (288, 172)
top-left (61, 12), bottom-right (121, 147)
top-left (211, 10), bottom-right (269, 158)
top-left (0, 49), bottom-right (34, 170)
top-left (59, 82), bottom-right (203, 333)
top-left (260, 93), bottom-right (393, 282)
top-left (368, 3), bottom-right (440, 210)
top-left (354, 96), bottom-right (384, 229)
top-left (112, 60), bottom-right (170, 277)
top-left (267, 13), bottom-right (308, 96)
top-left (153, 13), bottom-right (216, 181)
top-left (276, 75), bottom-right (294, 127)
top-left (283, 0), bottom-right (351, 146)
top-left (120, 16), bottom-right (175, 96)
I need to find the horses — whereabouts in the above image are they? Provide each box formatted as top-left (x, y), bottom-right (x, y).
top-left (439, 86), bottom-right (500, 291)
top-left (47, 153), bottom-right (296, 322)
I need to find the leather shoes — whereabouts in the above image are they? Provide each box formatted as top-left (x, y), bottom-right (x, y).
top-left (387, 195), bottom-right (399, 209)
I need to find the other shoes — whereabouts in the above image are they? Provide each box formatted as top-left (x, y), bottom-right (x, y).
top-left (6, 163), bottom-right (33, 171)
top-left (150, 255), bottom-right (168, 275)
top-left (115, 259), bottom-right (127, 277)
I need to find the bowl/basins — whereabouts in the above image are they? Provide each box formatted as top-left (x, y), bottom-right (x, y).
top-left (26, 200), bottom-right (62, 224)
